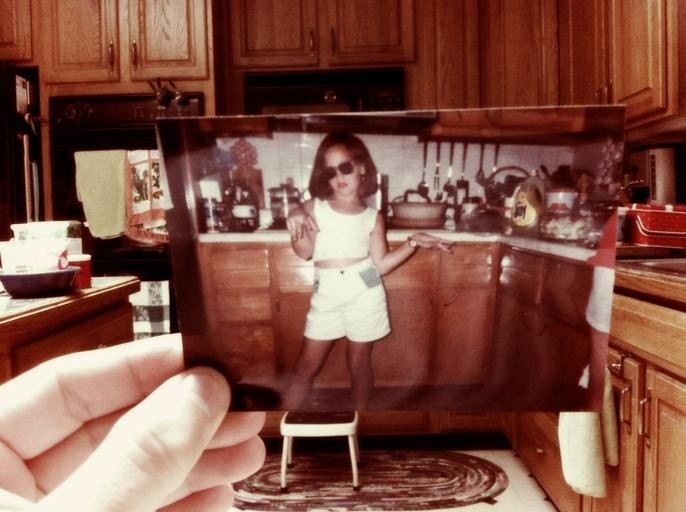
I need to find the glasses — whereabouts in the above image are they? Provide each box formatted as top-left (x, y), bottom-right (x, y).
top-left (323, 159), bottom-right (361, 179)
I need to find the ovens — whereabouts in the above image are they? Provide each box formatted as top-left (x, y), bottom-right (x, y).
top-left (54, 98), bottom-right (201, 253)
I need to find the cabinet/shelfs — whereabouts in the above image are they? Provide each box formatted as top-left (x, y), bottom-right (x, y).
top-left (194, 228), bottom-right (686, 512)
top-left (223, 0), bottom-right (417, 71)
top-left (35, 1), bottom-right (215, 97)
top-left (403, 0), bottom-right (686, 148)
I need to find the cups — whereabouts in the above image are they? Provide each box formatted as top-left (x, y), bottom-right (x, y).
top-left (64, 251), bottom-right (93, 291)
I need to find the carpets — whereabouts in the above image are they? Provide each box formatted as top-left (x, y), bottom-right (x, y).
top-left (229, 448), bottom-right (509, 512)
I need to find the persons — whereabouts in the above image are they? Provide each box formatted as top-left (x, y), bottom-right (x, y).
top-left (278, 130), bottom-right (457, 411)
top-left (0, 331), bottom-right (267, 512)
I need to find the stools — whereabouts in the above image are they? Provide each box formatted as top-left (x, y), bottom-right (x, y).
top-left (279, 410), bottom-right (362, 490)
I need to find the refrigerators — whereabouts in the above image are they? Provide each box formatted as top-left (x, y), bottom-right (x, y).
top-left (0, 66), bottom-right (43, 241)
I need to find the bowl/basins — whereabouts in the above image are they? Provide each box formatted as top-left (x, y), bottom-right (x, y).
top-left (390, 201), bottom-right (447, 228)
top-left (0, 266), bottom-right (82, 298)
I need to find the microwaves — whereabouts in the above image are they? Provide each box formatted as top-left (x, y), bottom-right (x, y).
top-left (245, 79), bottom-right (401, 116)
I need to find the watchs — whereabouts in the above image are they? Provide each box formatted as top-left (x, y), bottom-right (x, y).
top-left (406, 236), bottom-right (417, 248)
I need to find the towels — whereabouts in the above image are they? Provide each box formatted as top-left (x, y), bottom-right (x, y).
top-left (71, 149), bottom-right (169, 246)
